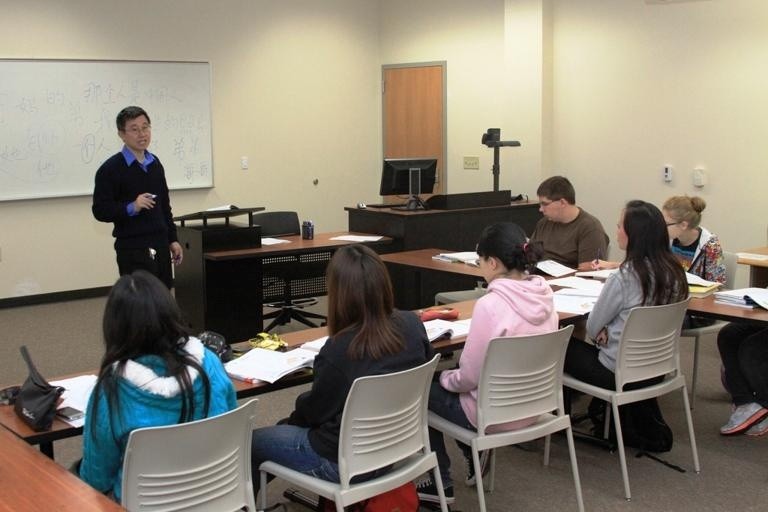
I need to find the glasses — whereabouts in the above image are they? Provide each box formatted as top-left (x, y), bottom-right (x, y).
top-left (122, 124), bottom-right (154, 133)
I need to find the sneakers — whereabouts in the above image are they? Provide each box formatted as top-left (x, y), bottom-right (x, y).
top-left (464, 448), bottom-right (495, 486)
top-left (417, 480), bottom-right (457, 506)
top-left (717, 403), bottom-right (764, 433)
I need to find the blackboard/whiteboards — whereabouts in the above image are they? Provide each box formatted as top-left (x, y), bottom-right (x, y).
top-left (0, 57), bottom-right (215, 202)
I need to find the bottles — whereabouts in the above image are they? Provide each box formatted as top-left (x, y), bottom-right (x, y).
top-left (303, 220), bottom-right (315, 240)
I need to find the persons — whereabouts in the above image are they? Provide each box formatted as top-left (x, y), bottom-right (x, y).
top-left (564, 200), bottom-right (689, 415)
top-left (529, 177), bottom-right (609, 269)
top-left (717, 319), bottom-right (768, 438)
top-left (68, 269), bottom-right (237, 504)
top-left (92, 106), bottom-right (183, 291)
top-left (416, 222), bottom-right (559, 506)
top-left (251, 244), bottom-right (436, 498)
top-left (662, 196), bottom-right (725, 329)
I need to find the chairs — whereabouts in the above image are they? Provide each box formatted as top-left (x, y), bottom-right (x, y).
top-left (681, 252), bottom-right (738, 411)
top-left (422, 324), bottom-right (584, 512)
top-left (121, 399), bottom-right (257, 512)
top-left (561, 295), bottom-right (701, 502)
top-left (252, 211), bottom-right (329, 334)
top-left (258, 352), bottom-right (449, 512)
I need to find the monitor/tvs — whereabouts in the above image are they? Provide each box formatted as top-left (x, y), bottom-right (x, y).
top-left (380, 158), bottom-right (438, 211)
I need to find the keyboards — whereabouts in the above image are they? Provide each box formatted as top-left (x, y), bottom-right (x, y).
top-left (366, 202), bottom-right (407, 208)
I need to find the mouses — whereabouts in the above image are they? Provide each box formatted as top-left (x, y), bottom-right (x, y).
top-left (357, 201), bottom-right (367, 209)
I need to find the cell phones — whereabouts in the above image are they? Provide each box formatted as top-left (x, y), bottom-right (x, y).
top-left (57, 405), bottom-right (84, 421)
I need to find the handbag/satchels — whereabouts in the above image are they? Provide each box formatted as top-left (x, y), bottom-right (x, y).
top-left (587, 384), bottom-right (674, 455)
top-left (13, 340), bottom-right (66, 432)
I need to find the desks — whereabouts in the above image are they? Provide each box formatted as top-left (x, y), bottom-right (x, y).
top-left (0, 424), bottom-right (133, 512)
top-left (375, 247), bottom-right (768, 329)
top-left (169, 207), bottom-right (393, 348)
top-left (1, 291), bottom-right (590, 463)
top-left (731, 246), bottom-right (768, 288)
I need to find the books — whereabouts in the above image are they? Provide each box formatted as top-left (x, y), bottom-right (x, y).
top-left (685, 271), bottom-right (768, 311)
top-left (576, 269), bottom-right (618, 278)
top-left (222, 334), bottom-right (329, 384)
top-left (432, 251), bottom-right (480, 267)
top-left (423, 318), bottom-right (471, 344)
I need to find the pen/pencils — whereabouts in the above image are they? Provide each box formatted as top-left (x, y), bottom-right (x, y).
top-left (596, 248), bottom-right (601, 271)
top-left (146, 194), bottom-right (157, 199)
top-left (55, 413), bottom-right (70, 419)
